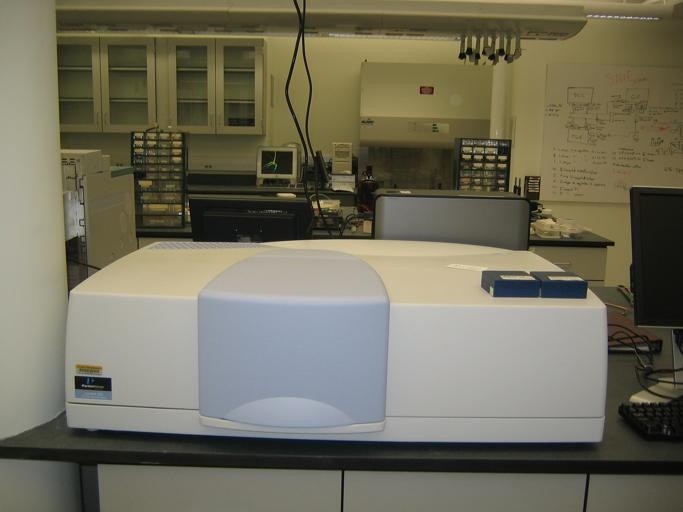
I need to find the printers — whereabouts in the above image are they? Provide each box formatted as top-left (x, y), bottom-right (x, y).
top-left (65, 240), bottom-right (608, 445)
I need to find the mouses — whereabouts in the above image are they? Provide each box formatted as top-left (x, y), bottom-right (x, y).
top-left (308, 185), bottom-right (315, 191)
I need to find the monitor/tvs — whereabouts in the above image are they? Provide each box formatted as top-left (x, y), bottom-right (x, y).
top-left (189, 195), bottom-right (314, 242)
top-left (628, 186), bottom-right (683, 403)
top-left (256, 147), bottom-right (297, 183)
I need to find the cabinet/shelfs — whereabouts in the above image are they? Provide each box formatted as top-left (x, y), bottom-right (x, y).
top-left (55, 35), bottom-right (156, 134)
top-left (156, 37), bottom-right (266, 136)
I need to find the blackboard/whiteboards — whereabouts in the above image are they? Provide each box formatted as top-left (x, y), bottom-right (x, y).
top-left (538, 63), bottom-right (683, 204)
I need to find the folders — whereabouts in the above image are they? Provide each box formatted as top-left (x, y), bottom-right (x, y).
top-left (607, 313), bottom-right (663, 354)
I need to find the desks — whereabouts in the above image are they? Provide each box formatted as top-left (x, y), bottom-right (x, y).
top-left (529, 228), bottom-right (615, 287)
top-left (0, 287), bottom-right (683, 512)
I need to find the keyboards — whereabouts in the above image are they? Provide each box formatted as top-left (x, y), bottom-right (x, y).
top-left (618, 402), bottom-right (683, 442)
top-left (255, 183), bottom-right (306, 193)
